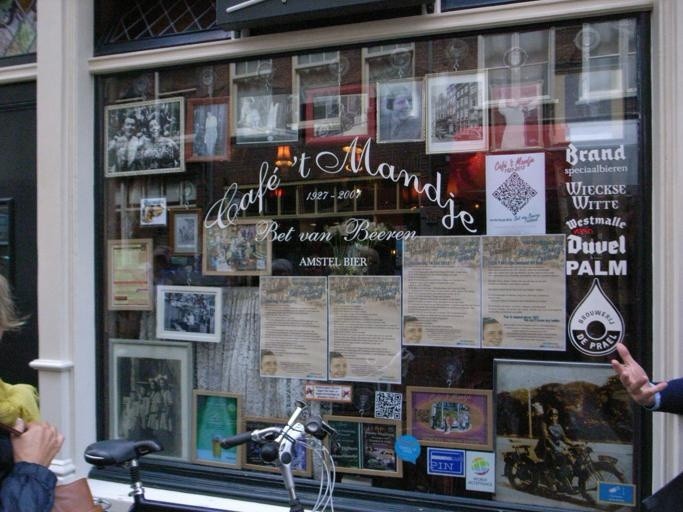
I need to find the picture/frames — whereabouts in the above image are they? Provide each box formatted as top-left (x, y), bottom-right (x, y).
top-left (155, 285), bottom-right (223, 343)
top-left (192, 389), bottom-right (243, 470)
top-left (305, 82), bottom-right (376, 144)
top-left (546, 56), bottom-right (629, 150)
top-left (490, 358), bottom-right (642, 512)
top-left (235, 89), bottom-right (305, 149)
top-left (321, 414), bottom-right (404, 478)
top-left (488, 79), bottom-right (543, 154)
top-left (201, 218), bottom-right (272, 276)
top-left (425, 68), bottom-right (489, 154)
top-left (376, 76), bottom-right (427, 144)
top-left (106, 239), bottom-right (154, 311)
top-left (168, 208), bottom-right (203, 257)
top-left (107, 339), bottom-right (194, 464)
top-left (104, 96), bottom-right (186, 178)
top-left (241, 415), bottom-right (313, 479)
top-left (185, 96), bottom-right (232, 161)
top-left (405, 386), bottom-right (495, 452)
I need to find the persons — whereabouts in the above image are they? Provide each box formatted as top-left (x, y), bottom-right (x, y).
top-left (106, 113), bottom-right (144, 173)
top-left (612, 341), bottom-right (682, 511)
top-left (497, 83), bottom-right (538, 151)
top-left (482, 316), bottom-right (503, 348)
top-left (121, 373), bottom-right (176, 454)
top-left (329, 351), bottom-right (348, 377)
top-left (0, 379), bottom-right (105, 512)
top-left (201, 110), bottom-right (218, 155)
top-left (384, 85), bottom-right (420, 140)
top-left (532, 407), bottom-right (585, 498)
top-left (404, 316), bottom-right (422, 345)
top-left (260, 350), bottom-right (277, 376)
top-left (135, 115), bottom-right (179, 171)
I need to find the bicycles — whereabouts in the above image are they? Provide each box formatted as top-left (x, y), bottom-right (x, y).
top-left (84, 400), bottom-right (342, 512)
top-left (316, 111), bottom-right (354, 138)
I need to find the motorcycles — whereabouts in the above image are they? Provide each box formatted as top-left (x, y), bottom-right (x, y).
top-left (501, 442), bottom-right (627, 511)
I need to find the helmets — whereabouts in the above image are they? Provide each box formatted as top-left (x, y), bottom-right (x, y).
top-left (546, 407), bottom-right (559, 416)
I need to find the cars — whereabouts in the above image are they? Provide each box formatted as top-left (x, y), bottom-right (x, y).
top-left (332, 439), bottom-right (359, 466)
